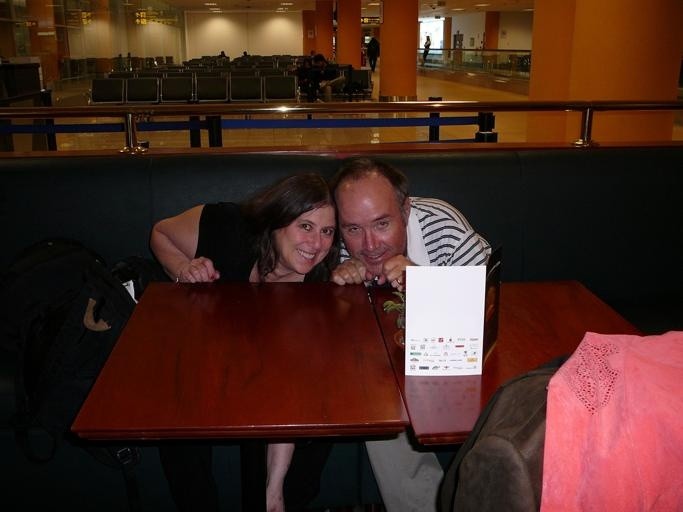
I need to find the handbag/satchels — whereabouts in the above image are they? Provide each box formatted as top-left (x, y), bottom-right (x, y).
top-left (84, 258), bottom-right (171, 344)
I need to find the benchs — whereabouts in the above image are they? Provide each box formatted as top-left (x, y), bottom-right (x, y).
top-left (89, 57), bottom-right (372, 104)
top-left (1, 145), bottom-right (683, 503)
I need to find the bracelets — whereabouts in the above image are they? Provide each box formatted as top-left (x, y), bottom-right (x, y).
top-left (176, 262), bottom-right (188, 285)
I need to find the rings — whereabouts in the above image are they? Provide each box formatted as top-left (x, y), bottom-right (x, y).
top-left (392, 280), bottom-right (400, 288)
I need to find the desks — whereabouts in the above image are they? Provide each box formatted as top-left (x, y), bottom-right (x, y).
top-left (367, 279), bottom-right (649, 470)
top-left (74, 281), bottom-right (408, 511)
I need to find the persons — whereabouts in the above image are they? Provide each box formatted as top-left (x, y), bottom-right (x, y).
top-left (150, 170), bottom-right (336, 512)
top-left (243, 51), bottom-right (250, 57)
top-left (220, 51), bottom-right (228, 59)
top-left (300, 58), bottom-right (318, 102)
top-left (116, 54), bottom-right (121, 69)
top-left (422, 36), bottom-right (431, 63)
top-left (335, 152), bottom-right (496, 512)
top-left (317, 54), bottom-right (347, 102)
top-left (126, 52), bottom-right (133, 68)
top-left (367, 38), bottom-right (382, 71)
